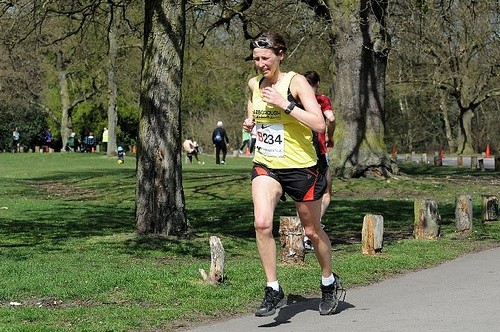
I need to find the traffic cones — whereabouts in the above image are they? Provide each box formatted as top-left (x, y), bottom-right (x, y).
top-left (133, 145), bottom-right (137, 153)
top-left (246, 147), bottom-right (251, 157)
top-left (391, 145), bottom-right (398, 158)
top-left (483, 144), bottom-right (493, 158)
top-left (440, 146), bottom-right (445, 158)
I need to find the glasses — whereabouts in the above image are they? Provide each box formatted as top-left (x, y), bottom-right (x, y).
top-left (250, 41), bottom-right (280, 50)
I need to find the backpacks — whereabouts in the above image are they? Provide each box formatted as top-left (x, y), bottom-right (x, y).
top-left (214, 128), bottom-right (223, 142)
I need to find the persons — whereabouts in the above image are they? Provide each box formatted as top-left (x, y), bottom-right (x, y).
top-left (241, 31), bottom-right (341, 317)
top-left (13, 123), bottom-right (257, 163)
top-left (212, 121), bottom-right (229, 164)
top-left (297, 67), bottom-right (336, 251)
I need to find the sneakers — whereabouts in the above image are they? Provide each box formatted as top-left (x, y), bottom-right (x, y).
top-left (318, 271), bottom-right (345, 315)
top-left (254, 285), bottom-right (285, 317)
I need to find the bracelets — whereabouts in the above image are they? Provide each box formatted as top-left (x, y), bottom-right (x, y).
top-left (284, 101), bottom-right (296, 114)
